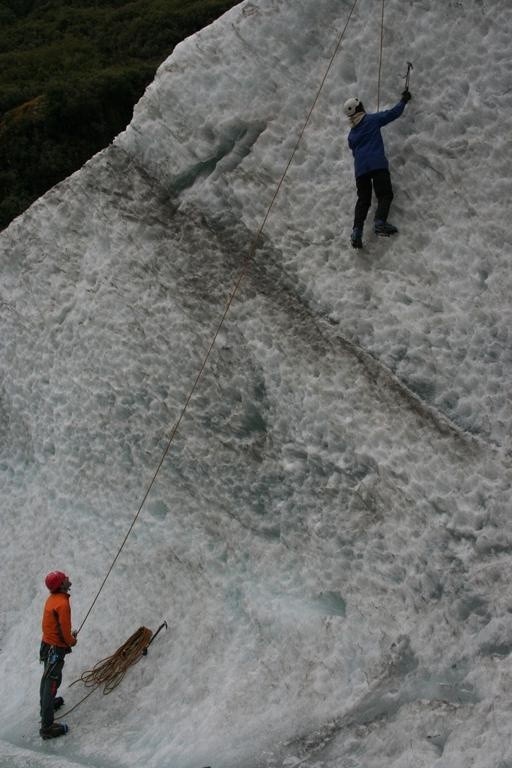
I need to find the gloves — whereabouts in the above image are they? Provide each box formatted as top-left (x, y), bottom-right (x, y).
top-left (402, 87), bottom-right (411, 101)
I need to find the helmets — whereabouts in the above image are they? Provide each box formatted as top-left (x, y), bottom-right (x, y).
top-left (45, 572), bottom-right (64, 592)
top-left (344, 98), bottom-right (360, 117)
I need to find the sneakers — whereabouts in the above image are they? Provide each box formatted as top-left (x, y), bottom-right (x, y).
top-left (350, 232), bottom-right (362, 248)
top-left (40, 723), bottom-right (68, 739)
top-left (375, 222), bottom-right (397, 234)
top-left (40, 697), bottom-right (63, 715)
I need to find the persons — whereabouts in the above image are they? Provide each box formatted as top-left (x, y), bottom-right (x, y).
top-left (341, 88), bottom-right (413, 249)
top-left (38, 569), bottom-right (80, 741)
